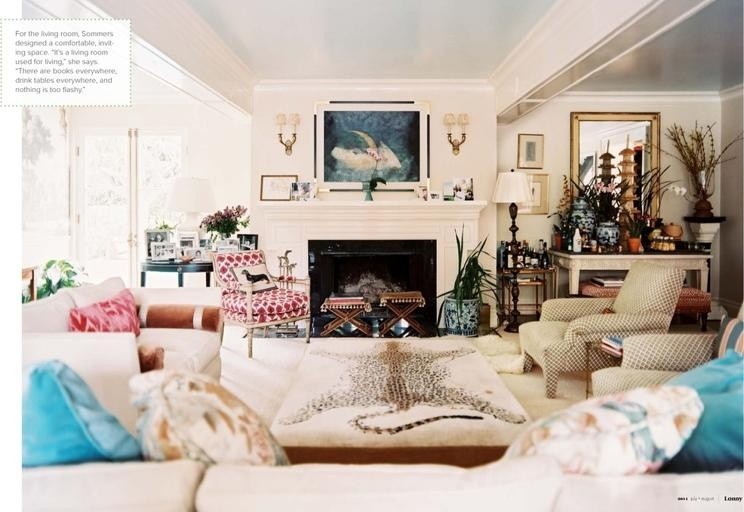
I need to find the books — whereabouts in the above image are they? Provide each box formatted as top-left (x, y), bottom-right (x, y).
top-left (600, 336), bottom-right (623, 356)
top-left (590, 276), bottom-right (625, 288)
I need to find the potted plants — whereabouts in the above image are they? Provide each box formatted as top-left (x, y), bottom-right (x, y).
top-left (627, 228), bottom-right (640, 254)
top-left (436, 224), bottom-right (508, 336)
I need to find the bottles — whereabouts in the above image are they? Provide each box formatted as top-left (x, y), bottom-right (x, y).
top-left (650, 235), bottom-right (676, 252)
top-left (498, 239), bottom-right (549, 270)
top-left (568, 227), bottom-right (583, 253)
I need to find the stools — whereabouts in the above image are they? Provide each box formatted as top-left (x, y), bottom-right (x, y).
top-left (580, 278), bottom-right (711, 331)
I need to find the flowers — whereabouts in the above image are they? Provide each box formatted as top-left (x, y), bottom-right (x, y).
top-left (200, 204), bottom-right (251, 239)
top-left (571, 172), bottom-right (631, 223)
top-left (365, 147), bottom-right (386, 187)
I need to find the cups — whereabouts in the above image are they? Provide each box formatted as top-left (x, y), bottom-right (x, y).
top-left (590, 239), bottom-right (606, 254)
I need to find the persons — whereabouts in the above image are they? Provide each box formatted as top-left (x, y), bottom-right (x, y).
top-left (154, 232), bottom-right (165, 242)
top-left (195, 250), bottom-right (202, 256)
top-left (243, 239), bottom-right (251, 251)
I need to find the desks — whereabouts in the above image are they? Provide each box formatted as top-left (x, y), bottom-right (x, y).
top-left (497, 266), bottom-right (558, 334)
top-left (140, 262), bottom-right (213, 288)
top-left (548, 249), bottom-right (713, 328)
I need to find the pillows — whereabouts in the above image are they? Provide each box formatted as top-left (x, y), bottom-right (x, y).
top-left (228, 264), bottom-right (277, 294)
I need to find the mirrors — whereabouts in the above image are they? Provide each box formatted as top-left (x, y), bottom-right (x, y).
top-left (570, 111), bottom-right (660, 224)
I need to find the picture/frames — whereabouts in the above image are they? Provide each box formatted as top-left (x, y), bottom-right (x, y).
top-left (260, 175), bottom-right (298, 201)
top-left (515, 133), bottom-right (550, 216)
top-left (144, 230), bottom-right (258, 265)
top-left (313, 100), bottom-right (430, 193)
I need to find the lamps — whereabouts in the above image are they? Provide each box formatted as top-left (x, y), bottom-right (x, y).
top-left (443, 113), bottom-right (471, 155)
top-left (276, 113), bottom-right (299, 155)
top-left (492, 169), bottom-right (533, 333)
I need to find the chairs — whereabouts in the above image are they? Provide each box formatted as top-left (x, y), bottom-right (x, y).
top-left (518, 260), bottom-right (688, 400)
top-left (211, 249), bottom-right (311, 358)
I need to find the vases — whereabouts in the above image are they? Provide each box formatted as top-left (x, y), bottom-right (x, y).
top-left (361, 181), bottom-right (377, 201)
top-left (573, 196), bottom-right (597, 244)
top-left (693, 189), bottom-right (714, 219)
top-left (594, 222), bottom-right (620, 253)
